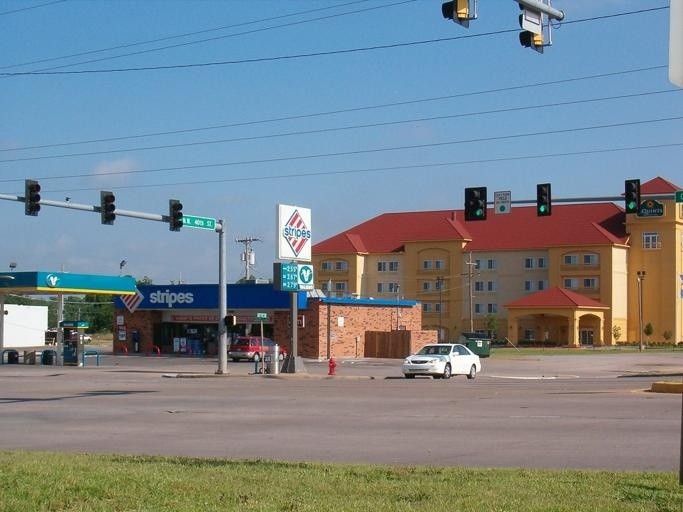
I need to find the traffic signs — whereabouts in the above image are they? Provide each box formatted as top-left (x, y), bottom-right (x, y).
top-left (279, 263), bottom-right (313, 291)
top-left (184, 214), bottom-right (217, 231)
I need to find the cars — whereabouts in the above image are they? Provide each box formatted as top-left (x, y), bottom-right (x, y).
top-left (73, 331), bottom-right (92, 345)
top-left (401, 343), bottom-right (482, 380)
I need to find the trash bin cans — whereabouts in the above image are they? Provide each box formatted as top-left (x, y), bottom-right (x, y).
top-left (269, 344), bottom-right (280, 374)
top-left (468, 338), bottom-right (490, 357)
top-left (8, 352), bottom-right (18, 364)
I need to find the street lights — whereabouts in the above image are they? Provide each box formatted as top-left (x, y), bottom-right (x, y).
top-left (636, 269), bottom-right (647, 350)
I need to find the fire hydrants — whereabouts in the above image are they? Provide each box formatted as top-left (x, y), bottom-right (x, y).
top-left (328, 358), bottom-right (337, 375)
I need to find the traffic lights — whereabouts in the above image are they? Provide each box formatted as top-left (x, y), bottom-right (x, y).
top-left (465, 186), bottom-right (488, 220)
top-left (169, 198), bottom-right (184, 231)
top-left (624, 180), bottom-right (641, 213)
top-left (518, 0), bottom-right (544, 54)
top-left (100, 190), bottom-right (116, 226)
top-left (537, 183), bottom-right (551, 217)
top-left (441, 0), bottom-right (469, 29)
top-left (26, 180), bottom-right (42, 216)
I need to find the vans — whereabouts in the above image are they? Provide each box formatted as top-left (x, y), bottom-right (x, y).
top-left (228, 334), bottom-right (288, 362)
